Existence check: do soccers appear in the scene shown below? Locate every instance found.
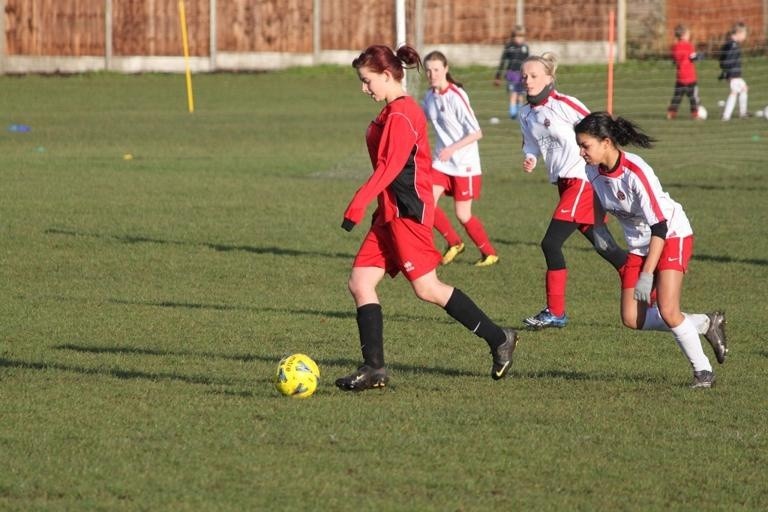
[763,105,768,118]
[274,354,321,397]
[698,105,707,119]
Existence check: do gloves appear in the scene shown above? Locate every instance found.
[632,272,653,305]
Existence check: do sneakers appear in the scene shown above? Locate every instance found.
[473,255,499,269]
[440,242,464,266]
[489,327,520,380]
[524,308,568,329]
[336,367,389,393]
[691,370,716,388]
[704,310,728,364]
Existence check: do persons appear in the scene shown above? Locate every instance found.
[333,44,520,390]
[574,111,727,389]
[416,50,500,266]
[717,22,755,119]
[494,26,529,119]
[666,22,701,119]
[519,53,628,328]
